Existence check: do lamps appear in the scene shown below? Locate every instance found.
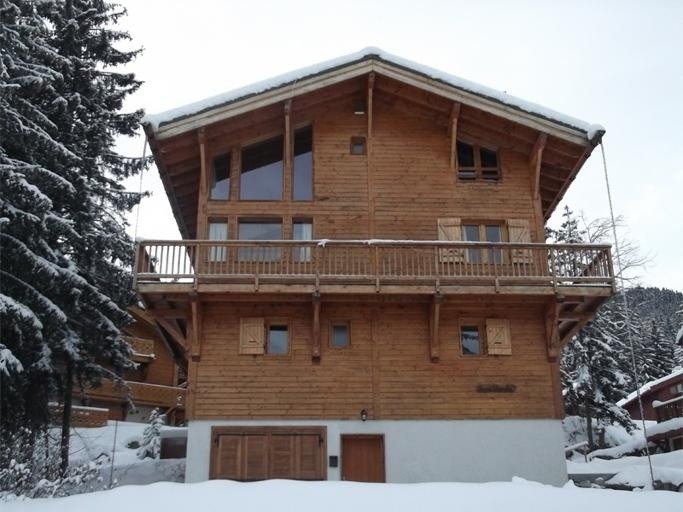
[360,409,369,422]
[312,289,320,301]
[351,78,365,115]
